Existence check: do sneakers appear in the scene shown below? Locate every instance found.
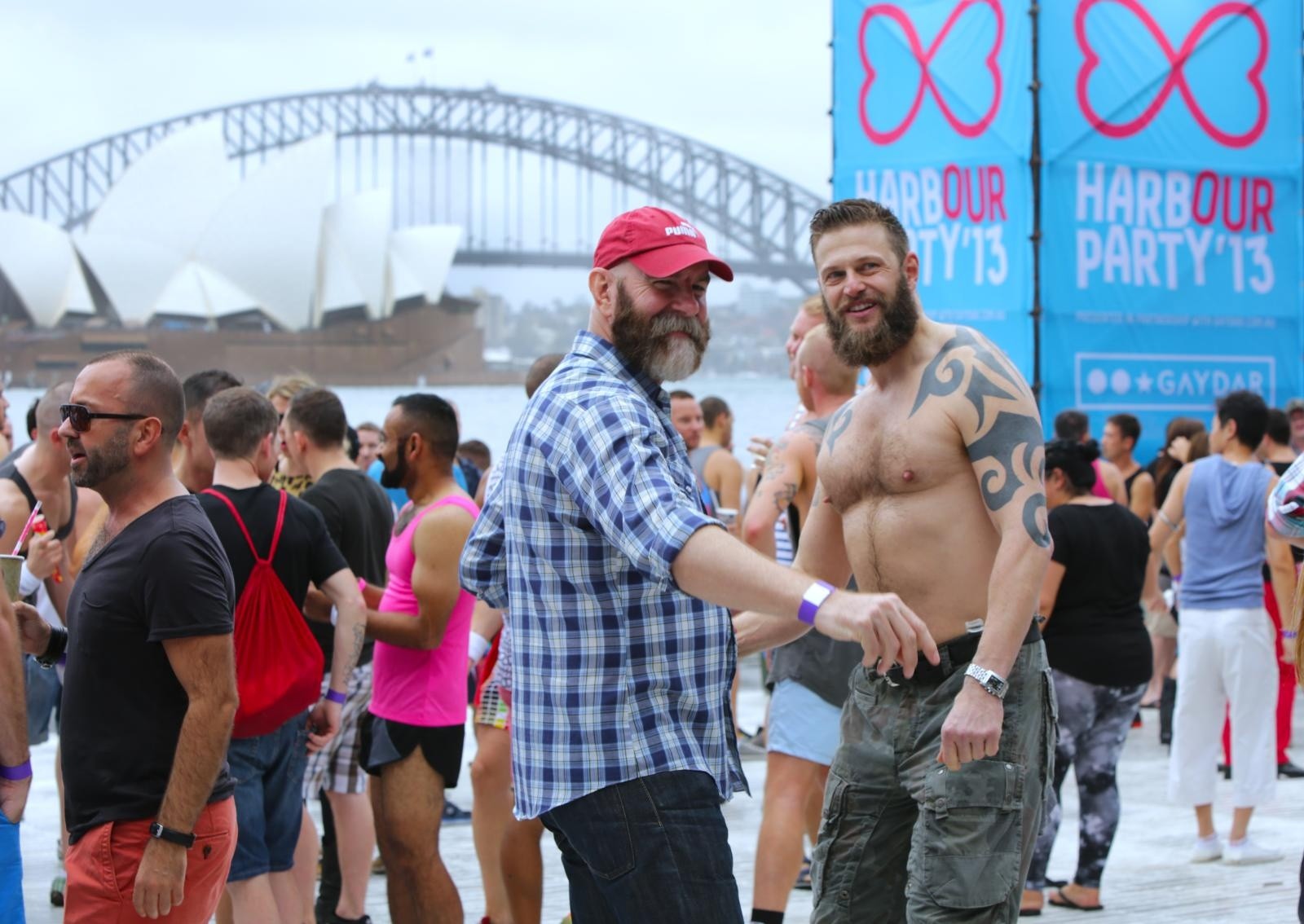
[1223,843,1285,865]
[1190,840,1223,861]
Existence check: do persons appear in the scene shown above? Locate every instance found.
[1053,392,1304,924]
[0,352,491,924]
[741,295,865,923]
[730,197,1059,924]
[459,206,941,924]
[1020,438,1153,916]
[469,354,745,924]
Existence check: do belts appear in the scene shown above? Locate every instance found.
[870,618,1044,688]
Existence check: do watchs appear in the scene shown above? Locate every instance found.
[797,578,837,627]
[964,663,1009,700]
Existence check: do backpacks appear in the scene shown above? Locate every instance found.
[198,487,323,737]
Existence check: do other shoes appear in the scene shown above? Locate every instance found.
[1278,764,1304,775]
[316,861,324,879]
[439,802,471,821]
[372,856,386,872]
[1218,765,1226,770]
[51,840,66,906]
[735,729,764,760]
[739,734,767,754]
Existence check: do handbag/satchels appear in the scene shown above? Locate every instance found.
[23,653,62,745]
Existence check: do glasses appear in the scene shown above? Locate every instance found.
[60,404,164,433]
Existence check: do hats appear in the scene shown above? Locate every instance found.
[1287,399,1304,415]
[594,206,734,282]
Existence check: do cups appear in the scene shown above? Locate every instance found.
[715,508,737,526]
[1,554,25,604]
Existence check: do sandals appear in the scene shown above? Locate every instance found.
[794,857,815,888]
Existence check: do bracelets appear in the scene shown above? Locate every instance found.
[326,687,346,702]
[330,599,338,627]
[1281,629,1297,639]
[1171,574,1181,580]
[3,759,32,780]
[358,575,367,592]
[35,618,66,669]
[148,821,197,850]
[18,560,42,595]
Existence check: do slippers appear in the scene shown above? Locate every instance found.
[1020,906,1040,914]
[1048,886,1103,909]
